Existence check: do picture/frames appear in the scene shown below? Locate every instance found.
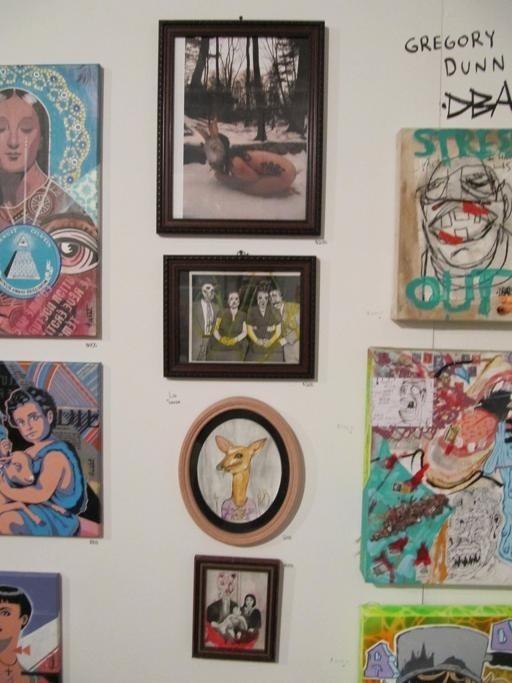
[163,254,318,382]
[156,18,325,236]
[177,396,306,548]
[191,555,283,663]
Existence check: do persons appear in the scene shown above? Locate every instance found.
[237,594,261,639]
[0,385,89,536]
[193,283,301,364]
[0,584,50,683]
[0,88,97,336]
[207,572,238,624]
